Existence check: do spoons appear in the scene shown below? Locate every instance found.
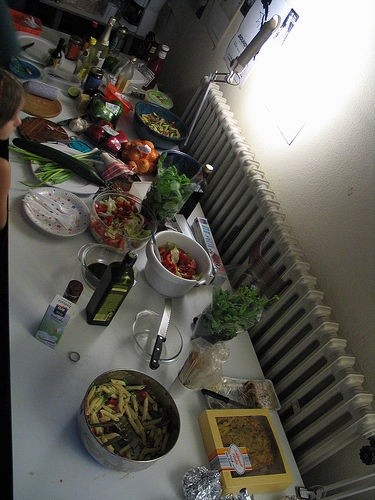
[15,56,33,76]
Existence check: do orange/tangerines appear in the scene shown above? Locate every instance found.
[120,140,160,174]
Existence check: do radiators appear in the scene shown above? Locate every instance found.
[177,76,374,473]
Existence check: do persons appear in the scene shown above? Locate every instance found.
[0,66,29,228]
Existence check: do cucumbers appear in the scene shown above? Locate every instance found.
[11,137,106,186]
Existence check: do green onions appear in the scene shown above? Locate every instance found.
[9,146,104,186]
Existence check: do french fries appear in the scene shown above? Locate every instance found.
[86,379,171,460]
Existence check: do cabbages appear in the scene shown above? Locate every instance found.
[95,195,152,242]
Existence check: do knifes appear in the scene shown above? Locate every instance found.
[201,386,253,408]
[148,294,173,368]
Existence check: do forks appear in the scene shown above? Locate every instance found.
[23,186,79,232]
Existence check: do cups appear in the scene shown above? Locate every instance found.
[88,65,104,93]
[171,337,230,392]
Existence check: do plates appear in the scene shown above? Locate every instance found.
[20,78,86,126]
[201,378,281,408]
[16,30,65,68]
[24,187,91,237]
[31,132,100,195]
[7,56,41,81]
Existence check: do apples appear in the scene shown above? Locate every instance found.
[87,119,128,152]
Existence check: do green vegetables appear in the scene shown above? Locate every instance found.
[196,285,280,345]
[143,156,191,221]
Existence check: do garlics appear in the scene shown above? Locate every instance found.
[69,118,90,131]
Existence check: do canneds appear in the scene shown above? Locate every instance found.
[84,66,105,96]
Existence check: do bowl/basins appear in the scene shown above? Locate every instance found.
[129,309,185,363]
[77,366,183,472]
[77,241,141,291]
[144,229,212,299]
[159,148,206,184]
[14,9,45,37]
[46,62,81,87]
[95,188,159,251]
[143,86,172,109]
[135,101,193,149]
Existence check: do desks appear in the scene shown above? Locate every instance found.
[9,25,309,500]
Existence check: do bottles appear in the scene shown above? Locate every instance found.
[142,43,171,91]
[110,54,140,88]
[95,16,117,70]
[180,160,213,222]
[80,35,99,67]
[85,250,139,326]
[47,36,67,69]
